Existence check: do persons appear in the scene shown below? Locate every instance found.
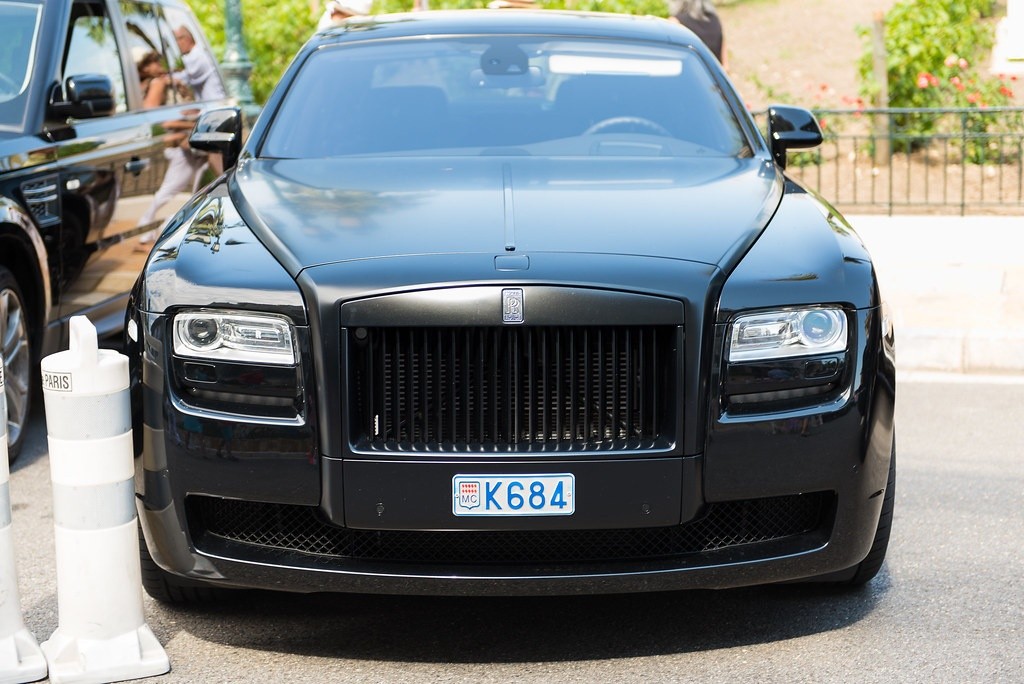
[670,0,723,65]
[137,26,244,245]
[317,0,373,29]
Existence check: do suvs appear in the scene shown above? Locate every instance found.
[0,0,242,471]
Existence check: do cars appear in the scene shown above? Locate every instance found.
[115,7,896,615]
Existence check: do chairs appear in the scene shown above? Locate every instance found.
[359,86,449,151]
[555,76,677,136]
